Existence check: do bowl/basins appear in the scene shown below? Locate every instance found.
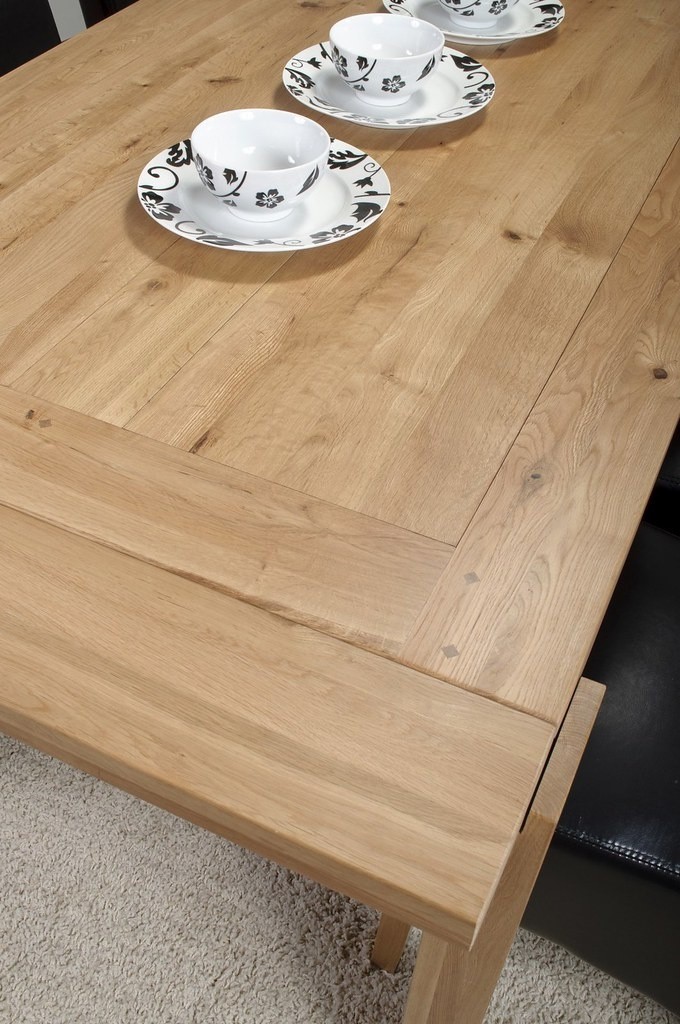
[328,14,445,107]
[438,0,512,29]
[192,108,330,222]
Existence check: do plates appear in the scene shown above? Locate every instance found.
[137,137,390,252]
[383,0,565,45]
[282,42,494,129]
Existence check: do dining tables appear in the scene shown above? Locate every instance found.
[0,0,680,1024]
